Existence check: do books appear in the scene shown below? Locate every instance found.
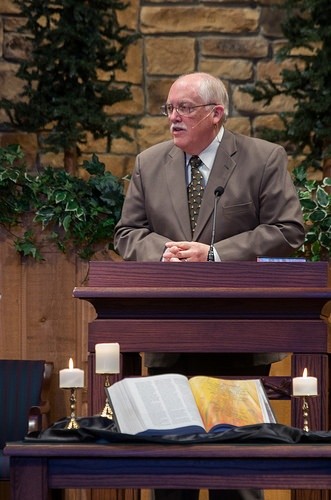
[104,372,277,434]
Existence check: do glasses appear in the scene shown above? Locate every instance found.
[159,102,218,116]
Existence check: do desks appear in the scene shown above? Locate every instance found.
[2,437,331,500]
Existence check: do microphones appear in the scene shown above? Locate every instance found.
[208,185,224,262]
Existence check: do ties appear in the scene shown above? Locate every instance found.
[186,155,204,237]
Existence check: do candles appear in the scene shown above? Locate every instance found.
[58,357,84,387]
[95,342,120,374]
[293,367,318,396]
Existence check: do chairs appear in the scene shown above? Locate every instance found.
[0,359,55,500]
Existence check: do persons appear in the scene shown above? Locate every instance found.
[113,72,306,500]
